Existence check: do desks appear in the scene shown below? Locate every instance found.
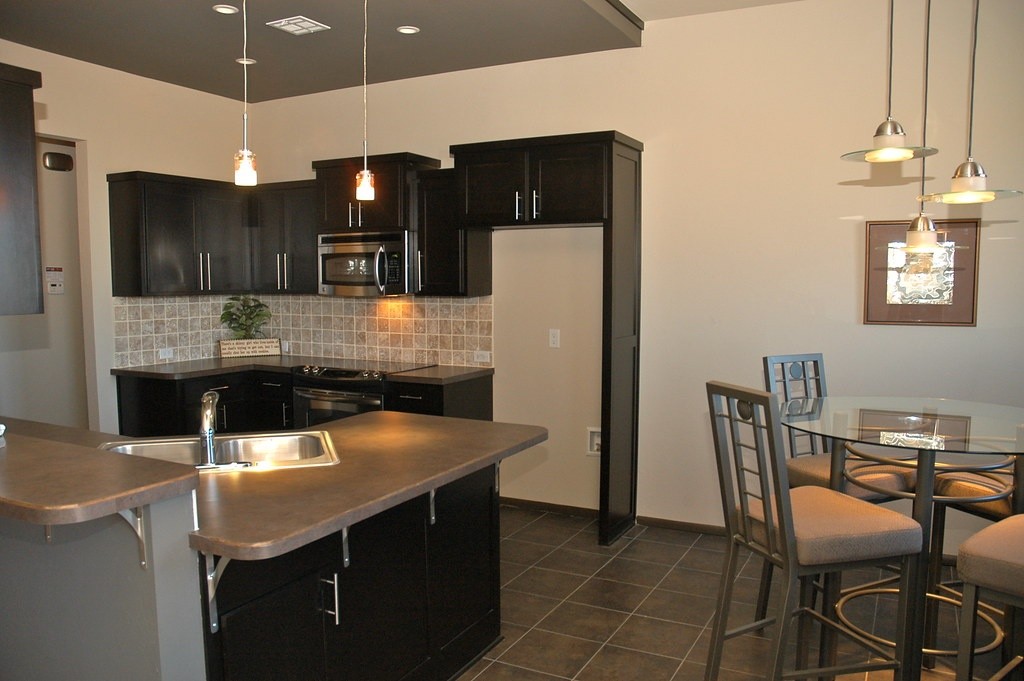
[777,396,1024,681]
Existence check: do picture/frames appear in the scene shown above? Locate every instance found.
[862,218,981,328]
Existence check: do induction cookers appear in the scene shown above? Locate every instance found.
[299,357,437,390]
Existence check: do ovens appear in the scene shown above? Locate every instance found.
[292,385,383,435]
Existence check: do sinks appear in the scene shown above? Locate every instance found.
[216,433,324,465]
[107,442,208,468]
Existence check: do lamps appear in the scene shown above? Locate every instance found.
[355,0,376,201]
[234,0,258,187]
[915,0,1024,205]
[840,0,938,164]
[875,0,970,254]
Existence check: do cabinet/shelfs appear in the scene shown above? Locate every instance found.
[248,179,317,294]
[0,61,44,315]
[116,371,255,437]
[385,374,493,422]
[256,370,295,431]
[312,152,441,234]
[448,130,644,230]
[199,460,505,681]
[415,168,492,299]
[107,171,250,297]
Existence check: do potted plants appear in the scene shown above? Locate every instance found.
[217,295,282,358]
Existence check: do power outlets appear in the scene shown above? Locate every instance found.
[283,342,289,352]
[549,328,560,348]
[160,348,173,360]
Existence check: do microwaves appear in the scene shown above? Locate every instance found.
[318,230,418,299]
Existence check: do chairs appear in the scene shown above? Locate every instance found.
[705,381,923,681]
[754,353,920,632]
[922,464,1015,670]
[956,512,1024,681]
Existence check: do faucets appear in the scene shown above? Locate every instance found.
[199,390,220,463]
